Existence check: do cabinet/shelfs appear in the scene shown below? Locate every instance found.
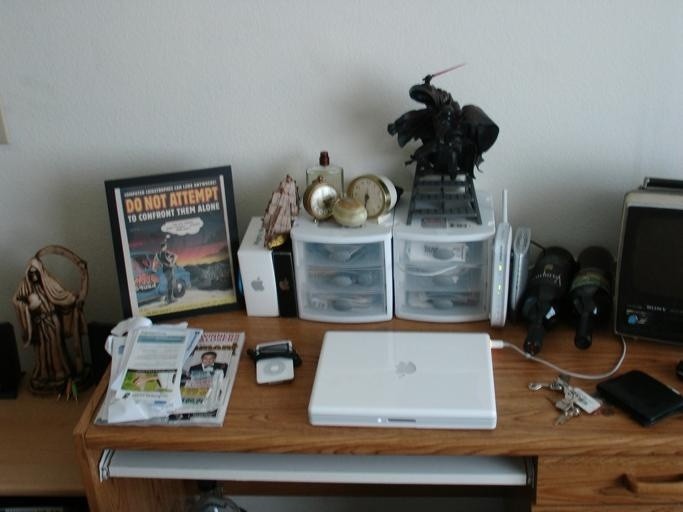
[291,204,391,322]
[393,189,491,323]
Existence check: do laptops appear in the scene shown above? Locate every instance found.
[308,330,497,430]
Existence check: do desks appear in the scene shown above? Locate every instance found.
[0,359,102,511]
[71,308,683,511]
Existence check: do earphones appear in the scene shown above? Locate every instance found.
[294,358,302,367]
[247,349,254,356]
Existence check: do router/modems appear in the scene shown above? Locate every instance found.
[489,189,512,328]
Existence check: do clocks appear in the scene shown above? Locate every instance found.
[346,175,398,217]
[303,174,338,222]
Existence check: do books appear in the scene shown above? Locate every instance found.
[97,327,245,427]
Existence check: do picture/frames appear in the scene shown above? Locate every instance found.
[105,165,243,322]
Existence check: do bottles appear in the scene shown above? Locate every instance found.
[570,246,619,350]
[306,152,344,199]
[523,246,577,356]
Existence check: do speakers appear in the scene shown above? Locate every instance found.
[0,322,22,400]
[613,188,683,348]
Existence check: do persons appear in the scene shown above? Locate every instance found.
[158,243,178,304]
[11,258,76,381]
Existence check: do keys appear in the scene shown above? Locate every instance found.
[545,374,605,424]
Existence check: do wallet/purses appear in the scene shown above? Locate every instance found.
[596,370,682,426]
